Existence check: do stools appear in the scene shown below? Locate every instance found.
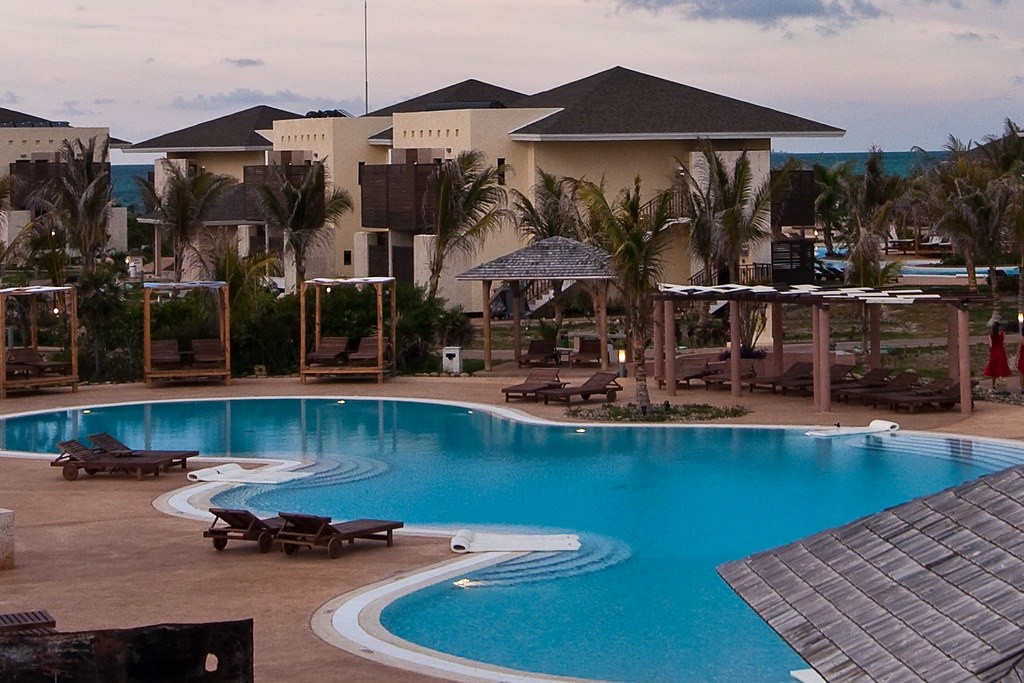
[0,610,56,632]
[0,627,57,634]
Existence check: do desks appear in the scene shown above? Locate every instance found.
[108,451,133,475]
[913,390,935,411]
[558,350,574,366]
[277,523,312,551]
[545,381,570,400]
[701,369,722,389]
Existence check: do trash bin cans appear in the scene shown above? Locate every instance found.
[442,346,463,373]
[129,265,137,278]
[560,330,568,348]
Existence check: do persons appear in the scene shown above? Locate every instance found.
[982,310,1013,390]
[1015,326,1024,395]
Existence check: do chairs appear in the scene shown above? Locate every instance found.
[814,266,903,283]
[151,340,180,370]
[502,367,561,402]
[986,270,1019,291]
[193,340,225,369]
[655,357,979,415]
[51,440,171,481]
[570,340,610,368]
[202,507,404,559]
[349,337,389,366]
[307,337,350,365]
[87,433,199,473]
[519,340,556,367]
[537,372,623,406]
[4,348,68,378]
